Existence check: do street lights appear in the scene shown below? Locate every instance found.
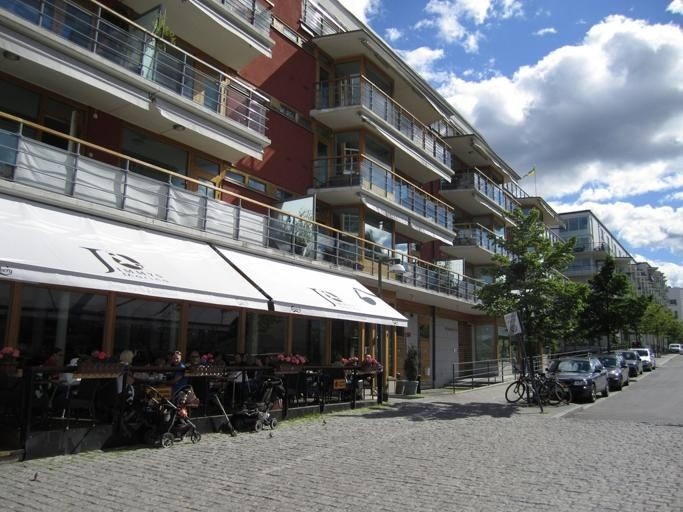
[377,257,405,404]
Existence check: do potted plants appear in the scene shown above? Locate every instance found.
[402,346,424,394]
[289,209,314,255]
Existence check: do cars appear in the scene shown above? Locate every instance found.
[670,344,680,353]
[552,347,655,403]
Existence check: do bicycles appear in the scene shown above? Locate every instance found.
[505,367,573,405]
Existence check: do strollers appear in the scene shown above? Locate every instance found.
[234,378,286,431]
[142,384,202,448]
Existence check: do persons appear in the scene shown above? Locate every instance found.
[24,338,272,414]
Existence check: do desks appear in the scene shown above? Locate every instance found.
[120,362,384,419]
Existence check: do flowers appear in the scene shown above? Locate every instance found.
[273,352,307,366]
[339,354,381,370]
